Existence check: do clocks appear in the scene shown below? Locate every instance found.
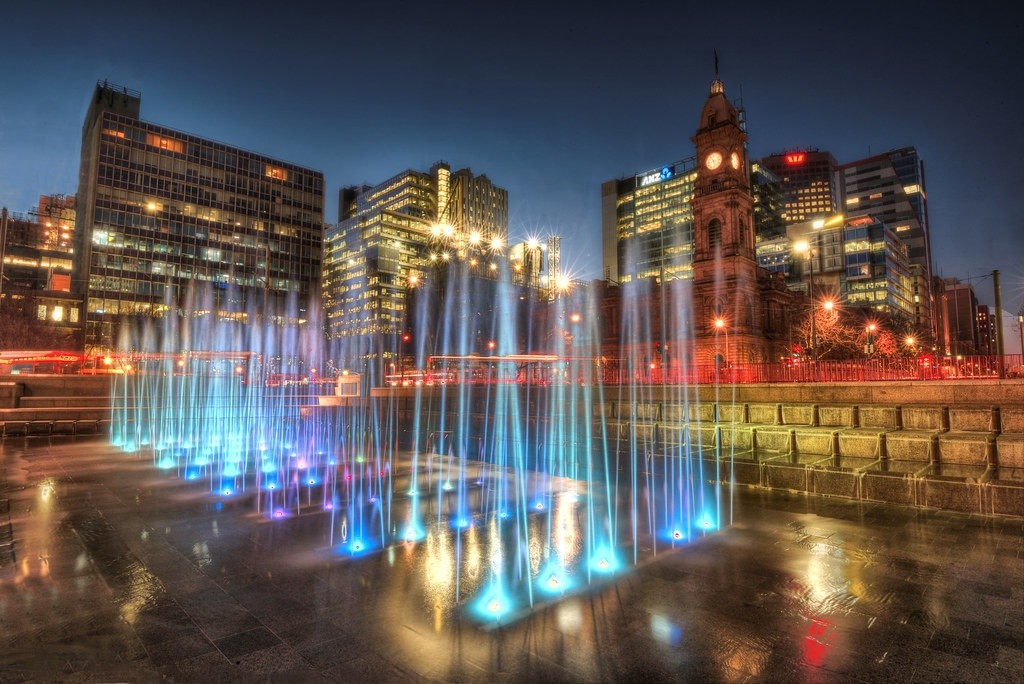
[729,153,740,169]
[704,153,722,172]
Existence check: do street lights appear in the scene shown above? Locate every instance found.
[824,302,833,319]
[869,324,875,360]
[794,241,815,372]
[571,314,580,356]
[390,364,395,383]
[527,238,540,303]
[908,338,914,364]
[401,277,417,384]
[715,320,729,371]
